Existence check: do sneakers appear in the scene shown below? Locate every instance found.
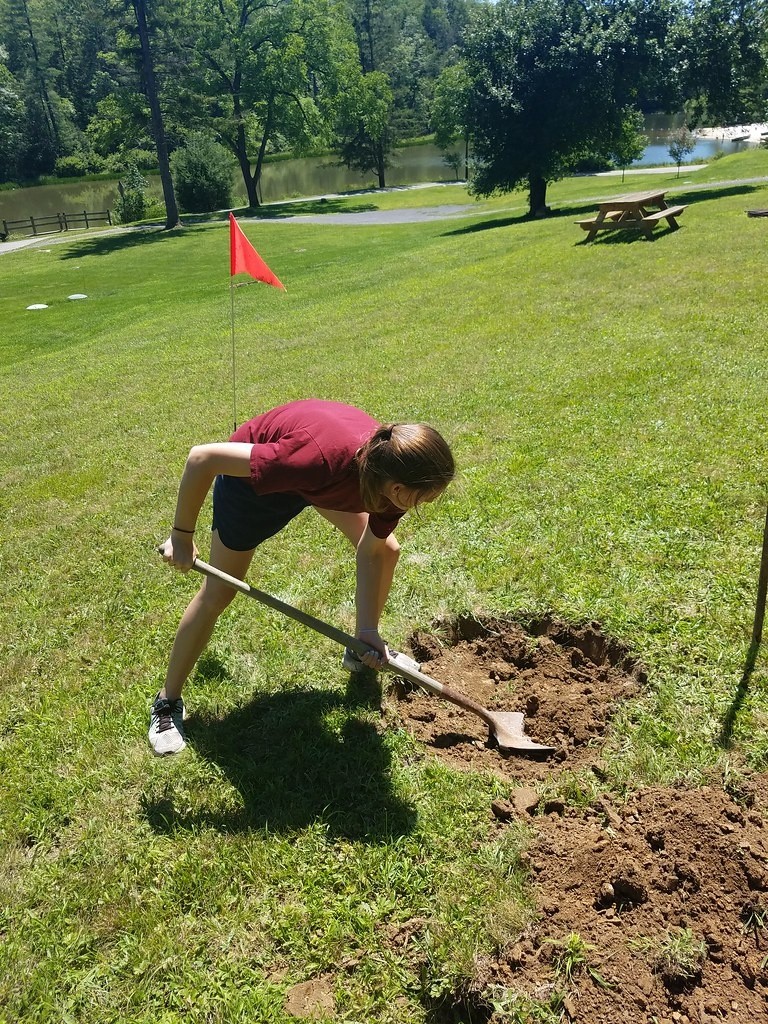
[342,643,422,673]
[148,691,187,756]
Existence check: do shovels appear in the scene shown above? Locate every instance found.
[158,538,560,754]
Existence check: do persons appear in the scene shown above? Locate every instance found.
[148,399,458,756]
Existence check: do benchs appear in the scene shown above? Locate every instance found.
[640,203,690,228]
[573,211,624,231]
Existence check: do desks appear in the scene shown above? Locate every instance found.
[586,190,669,244]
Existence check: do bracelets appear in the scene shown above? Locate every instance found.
[170,527,196,533]
[358,629,378,633]
